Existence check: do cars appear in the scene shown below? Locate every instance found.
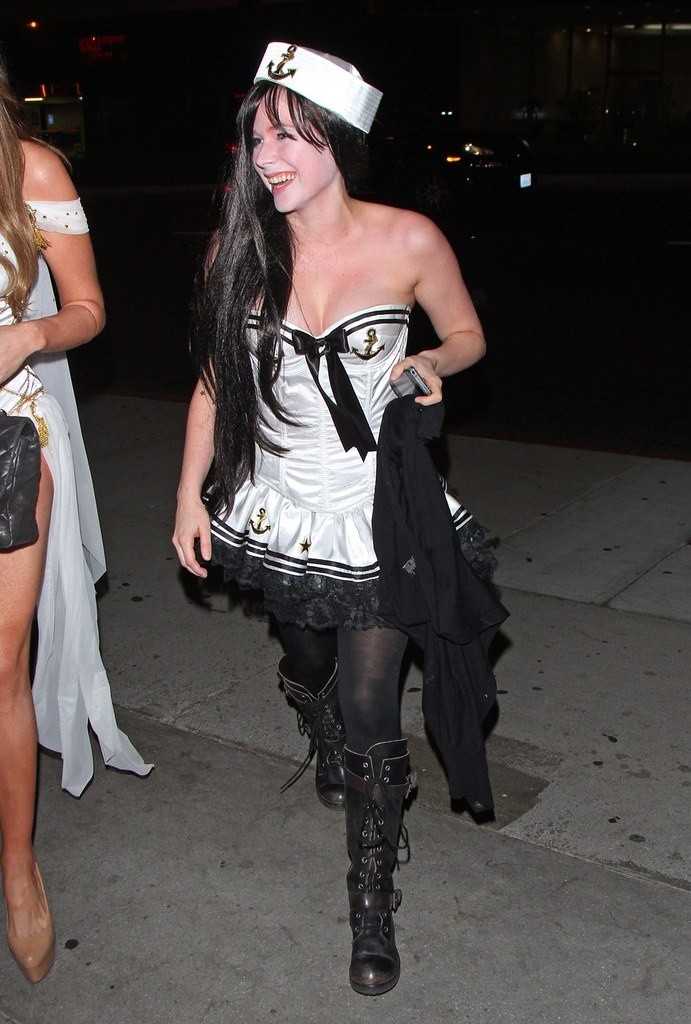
[339,91,541,219]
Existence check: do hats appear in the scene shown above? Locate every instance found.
[251,40,384,134]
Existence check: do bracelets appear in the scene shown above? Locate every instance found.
[58,304,98,346]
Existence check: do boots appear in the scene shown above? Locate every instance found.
[341,737,420,997]
[276,653,346,813]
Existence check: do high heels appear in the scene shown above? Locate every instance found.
[3,860,57,983]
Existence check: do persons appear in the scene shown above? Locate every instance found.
[0,71,110,983]
[168,40,488,998]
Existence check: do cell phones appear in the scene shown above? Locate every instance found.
[391,365,431,397]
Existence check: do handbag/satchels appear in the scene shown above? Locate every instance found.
[0,409,42,549]
